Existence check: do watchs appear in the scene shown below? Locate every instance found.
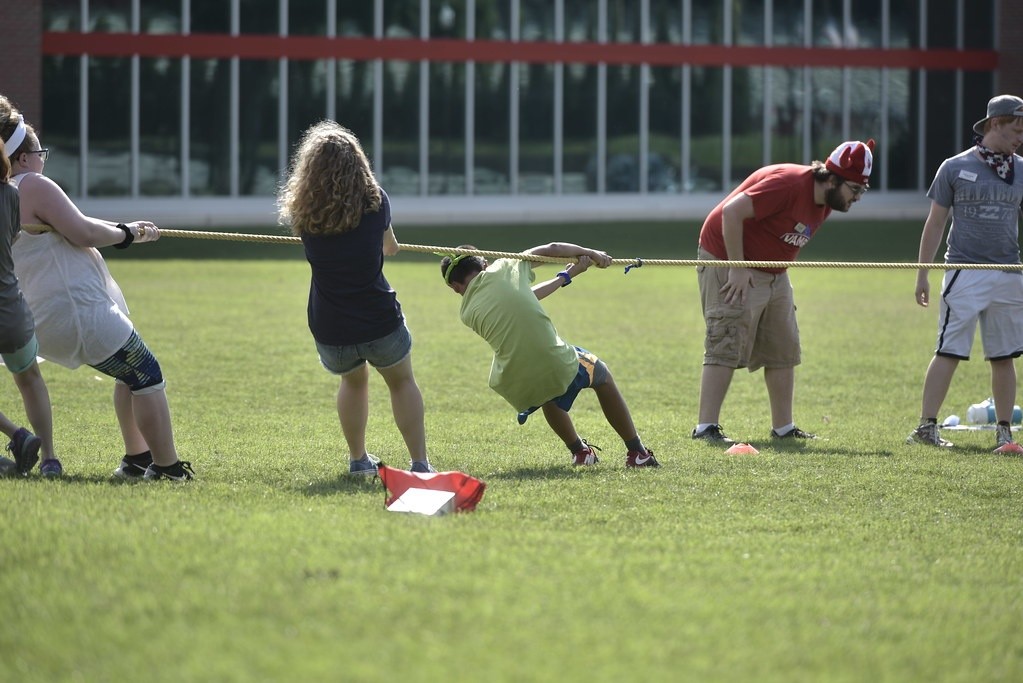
[557,271,573,288]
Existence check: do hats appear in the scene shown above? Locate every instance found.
[973,94,1023,136]
[825,139,875,185]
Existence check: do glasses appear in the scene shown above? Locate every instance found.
[16,148,49,160]
[845,182,868,195]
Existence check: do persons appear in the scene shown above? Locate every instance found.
[691,140,876,442]
[277,122,436,480]
[0,95,194,483]
[443,243,661,471]
[907,95,1023,448]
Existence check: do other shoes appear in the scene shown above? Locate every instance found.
[410,462,439,473]
[5,427,42,474]
[38,458,62,477]
[350,453,380,474]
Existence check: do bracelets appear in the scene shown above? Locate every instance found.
[113,223,134,250]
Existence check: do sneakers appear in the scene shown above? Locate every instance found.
[770,426,829,441]
[626,446,662,468]
[996,420,1013,446]
[692,424,735,443]
[573,439,602,465]
[906,417,953,447]
[114,458,195,481]
